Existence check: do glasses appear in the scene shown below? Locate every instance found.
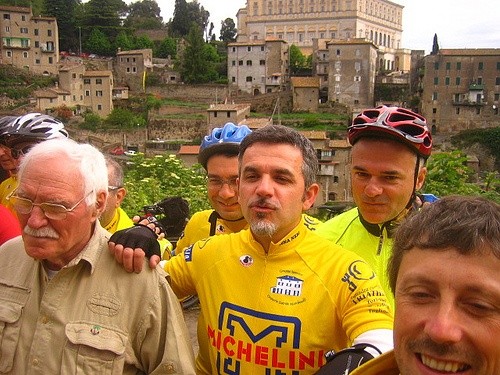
[11,145,31,158]
[6,188,93,220]
[108,186,122,192]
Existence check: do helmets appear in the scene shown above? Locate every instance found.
[196,123,254,170]
[8,113,69,143]
[348,105,432,159]
[0,116,17,134]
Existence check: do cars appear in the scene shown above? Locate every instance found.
[110,148,123,154]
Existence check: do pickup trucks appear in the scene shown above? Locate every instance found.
[125,150,138,155]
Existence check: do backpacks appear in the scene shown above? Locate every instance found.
[144,196,190,226]
